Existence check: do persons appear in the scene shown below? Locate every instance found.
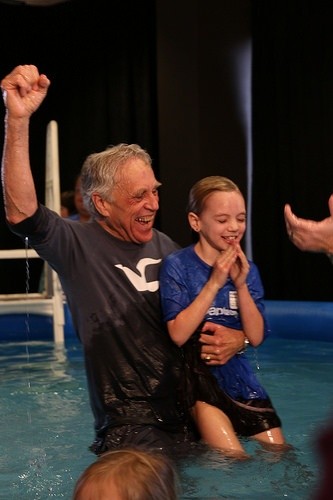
[37,193,76,334]
[0,65,251,434]
[284,194,333,254]
[160,176,286,449]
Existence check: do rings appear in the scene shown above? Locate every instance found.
[290,232,293,240]
[204,356,210,362]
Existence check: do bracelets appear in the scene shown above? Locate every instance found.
[237,337,249,354]
